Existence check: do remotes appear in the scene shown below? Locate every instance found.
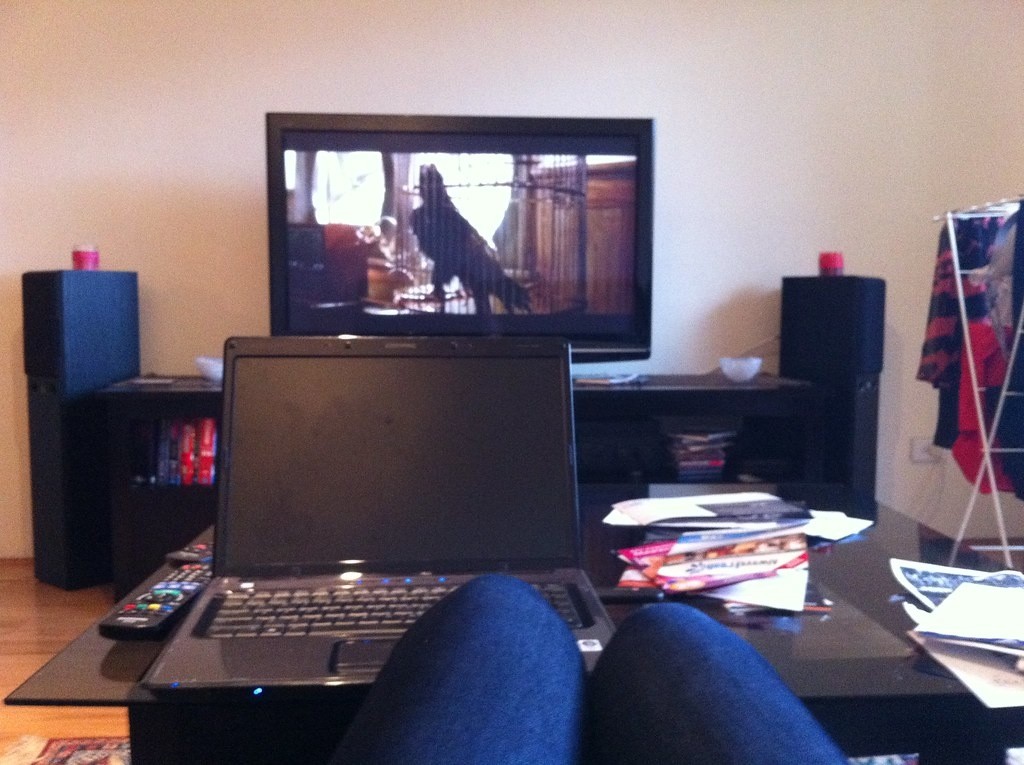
[98,538,215,643]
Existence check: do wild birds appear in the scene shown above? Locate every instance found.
[409,164,534,314]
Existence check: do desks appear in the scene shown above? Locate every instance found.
[0,481,1024,765]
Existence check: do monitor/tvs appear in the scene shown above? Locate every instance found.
[262,109,651,363]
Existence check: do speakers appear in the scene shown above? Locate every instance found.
[19,270,140,590]
[776,277,887,510]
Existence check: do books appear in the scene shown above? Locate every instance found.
[656,417,738,483]
[889,558,1024,709]
[604,493,876,616]
[572,373,638,385]
[154,417,219,485]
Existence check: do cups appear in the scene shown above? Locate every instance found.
[72,245,101,272]
[818,250,844,277]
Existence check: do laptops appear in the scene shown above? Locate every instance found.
[146,335,616,703]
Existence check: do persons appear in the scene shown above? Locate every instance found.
[326,572,852,765]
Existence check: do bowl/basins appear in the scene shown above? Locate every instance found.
[196,356,224,383]
[720,356,763,384]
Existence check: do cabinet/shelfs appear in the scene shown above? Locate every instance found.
[73,374,812,600]
